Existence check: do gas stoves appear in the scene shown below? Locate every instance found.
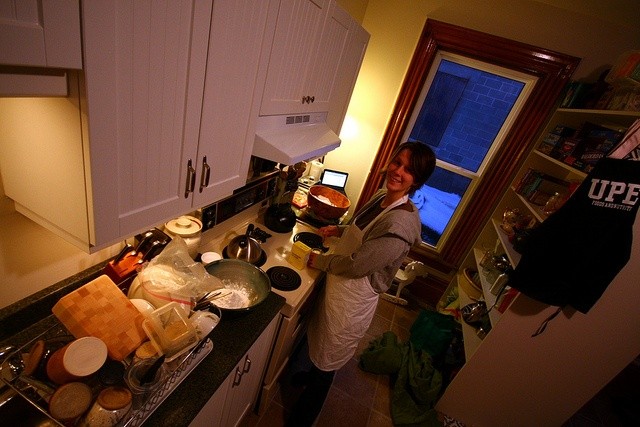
[202,179,340,307]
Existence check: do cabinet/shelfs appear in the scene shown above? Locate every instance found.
[258,283,318,416]
[2,0,84,97]
[81,0,271,248]
[188,316,279,427]
[259,0,354,116]
[435,111,640,427]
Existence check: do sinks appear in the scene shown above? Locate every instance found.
[1,386,49,426]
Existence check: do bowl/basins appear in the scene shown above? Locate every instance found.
[308,185,351,219]
[459,267,482,299]
[203,259,271,312]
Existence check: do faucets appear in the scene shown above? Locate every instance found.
[0,345,26,375]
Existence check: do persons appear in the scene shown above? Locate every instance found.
[284,141,436,426]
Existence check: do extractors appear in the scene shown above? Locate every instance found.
[251,111,342,166]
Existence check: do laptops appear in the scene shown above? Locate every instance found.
[322,168,349,198]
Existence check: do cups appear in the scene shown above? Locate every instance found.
[308,247,322,269]
[201,251,221,265]
[190,310,220,340]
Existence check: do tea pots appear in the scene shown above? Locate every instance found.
[227,224,263,263]
[263,191,296,233]
[461,301,486,324]
[502,206,523,232]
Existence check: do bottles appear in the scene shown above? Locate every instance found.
[479,250,495,266]
[482,256,497,275]
[486,262,507,284]
[489,271,508,296]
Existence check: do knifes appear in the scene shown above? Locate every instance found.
[113,232,160,267]
